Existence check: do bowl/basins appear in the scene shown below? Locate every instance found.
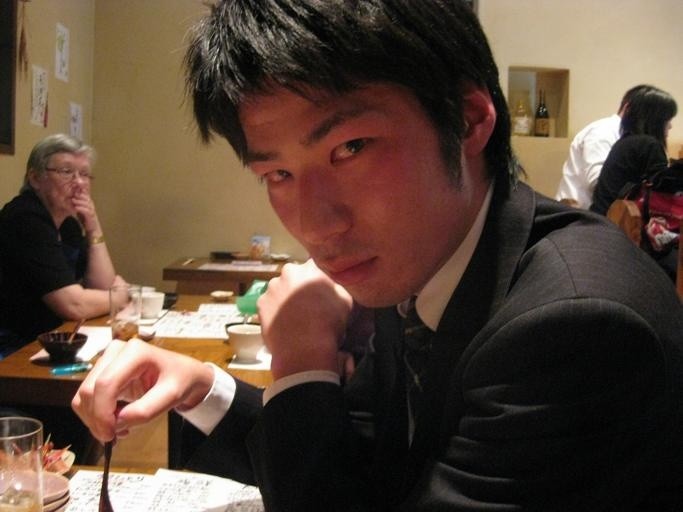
[210,290,234,302]
[163,293,178,308]
[37,333,87,362]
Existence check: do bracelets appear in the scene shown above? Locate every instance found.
[86,235,108,246]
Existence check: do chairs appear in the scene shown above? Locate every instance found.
[607,198,642,248]
[674,227,683,299]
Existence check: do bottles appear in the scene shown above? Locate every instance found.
[511,88,532,136]
[533,89,550,137]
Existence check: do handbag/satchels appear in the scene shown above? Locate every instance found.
[636,176,682,251]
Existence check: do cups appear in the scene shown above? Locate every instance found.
[0,417,44,512]
[227,322,262,364]
[133,292,165,318]
[140,286,155,293]
[111,285,142,340]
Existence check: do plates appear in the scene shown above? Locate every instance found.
[272,254,291,261]
[17,449,76,475]
[44,490,70,512]
[0,469,70,505]
[52,497,69,512]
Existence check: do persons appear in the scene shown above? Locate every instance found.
[590,89,682,218]
[557,86,658,208]
[0,136,130,462]
[70,1,681,511]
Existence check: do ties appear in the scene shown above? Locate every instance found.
[400,299,429,455]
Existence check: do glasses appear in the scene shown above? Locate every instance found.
[41,165,96,187]
